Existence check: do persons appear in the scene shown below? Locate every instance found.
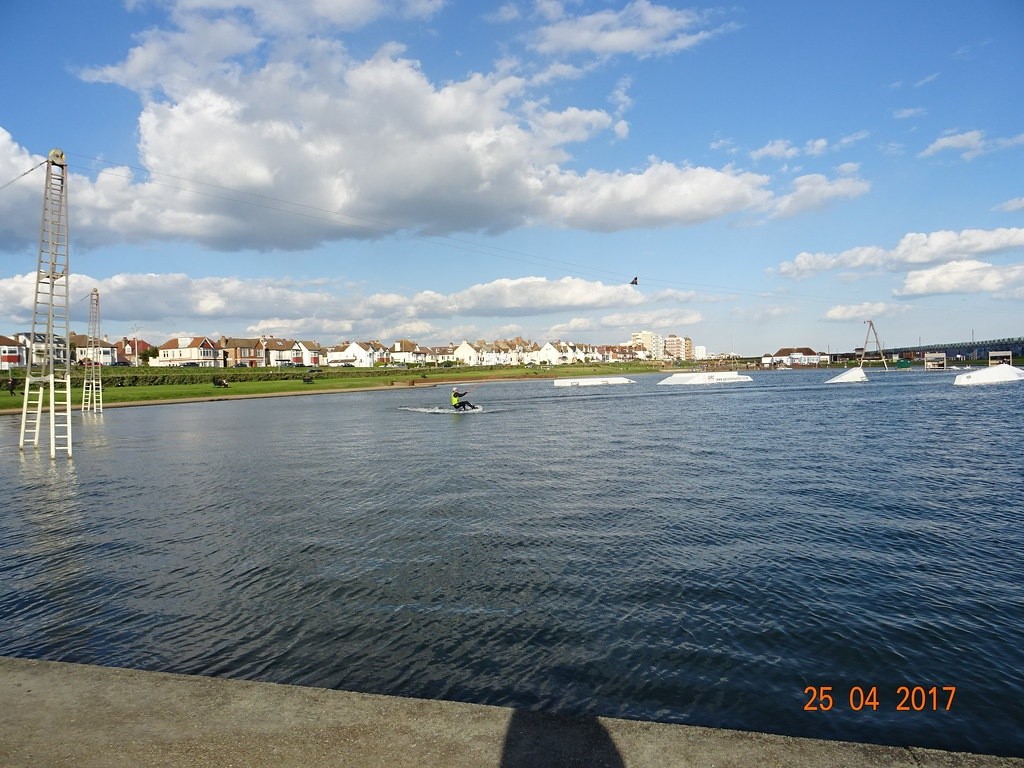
[451,387,478,411]
[223,378,229,388]
[7,378,16,396]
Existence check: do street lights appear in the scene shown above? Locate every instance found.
[402,336,411,357]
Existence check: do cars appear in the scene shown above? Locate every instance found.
[84,362,103,366]
[108,361,129,366]
[179,363,199,368]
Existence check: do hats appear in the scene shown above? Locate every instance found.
[452,387,458,391]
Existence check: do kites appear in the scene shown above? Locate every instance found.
[630,277,637,285]
